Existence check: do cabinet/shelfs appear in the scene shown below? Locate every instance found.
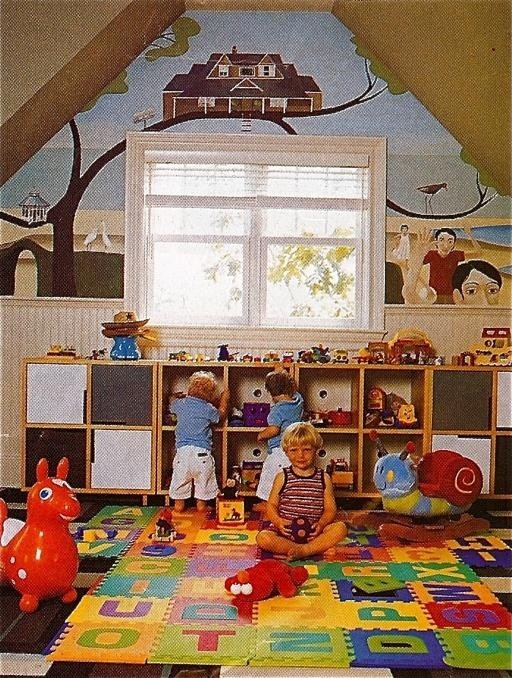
[18,357,512,508]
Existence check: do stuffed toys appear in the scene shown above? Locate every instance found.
[352,428,493,543]
[223,559,310,604]
[163,391,187,426]
[294,512,314,540]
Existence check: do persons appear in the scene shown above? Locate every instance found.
[256,420,347,561]
[402,226,502,305]
[166,371,230,515]
[390,224,413,271]
[424,226,486,305]
[256,370,305,502]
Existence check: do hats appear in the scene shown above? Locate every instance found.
[101,312,150,337]
